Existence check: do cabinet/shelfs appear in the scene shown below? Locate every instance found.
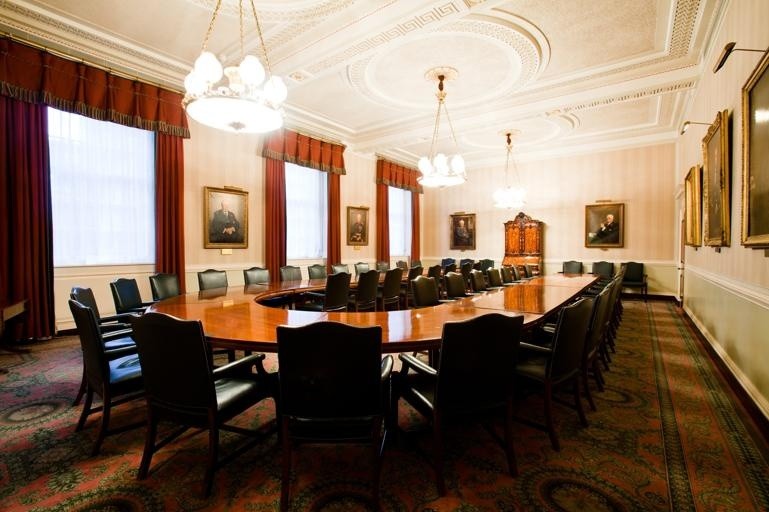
[500,213,545,278]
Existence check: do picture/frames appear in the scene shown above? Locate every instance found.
[446,210,476,253]
[681,46,769,260]
[582,200,626,251]
[201,185,250,251]
[345,204,371,247]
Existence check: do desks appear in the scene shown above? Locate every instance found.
[150,284,542,469]
[260,271,463,307]
[411,274,604,370]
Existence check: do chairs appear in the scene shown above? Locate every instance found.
[108,278,157,322]
[197,269,228,290]
[468,269,499,294]
[523,265,533,279]
[391,313,522,499]
[479,259,494,268]
[148,273,179,301]
[276,322,393,511]
[426,265,441,288]
[69,285,130,405]
[377,268,404,312]
[460,259,474,268]
[544,288,611,414]
[69,300,149,452]
[460,262,471,293]
[128,313,282,487]
[486,267,512,288]
[513,266,522,280]
[442,257,456,269]
[399,265,424,310]
[299,272,352,312]
[446,272,481,300]
[243,267,283,308]
[569,264,628,365]
[501,266,525,284]
[410,275,453,310]
[469,263,480,292]
[308,264,326,279]
[511,299,595,454]
[440,263,456,298]
[587,261,615,278]
[558,260,582,273]
[620,263,648,304]
[279,265,306,309]
[348,269,381,313]
[354,261,421,274]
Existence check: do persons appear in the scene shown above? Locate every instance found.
[592,212,618,243]
[456,220,469,245]
[209,197,240,243]
[350,212,364,240]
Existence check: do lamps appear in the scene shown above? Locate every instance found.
[712,41,767,73]
[490,128,529,212]
[181,0,288,138]
[414,64,467,188]
